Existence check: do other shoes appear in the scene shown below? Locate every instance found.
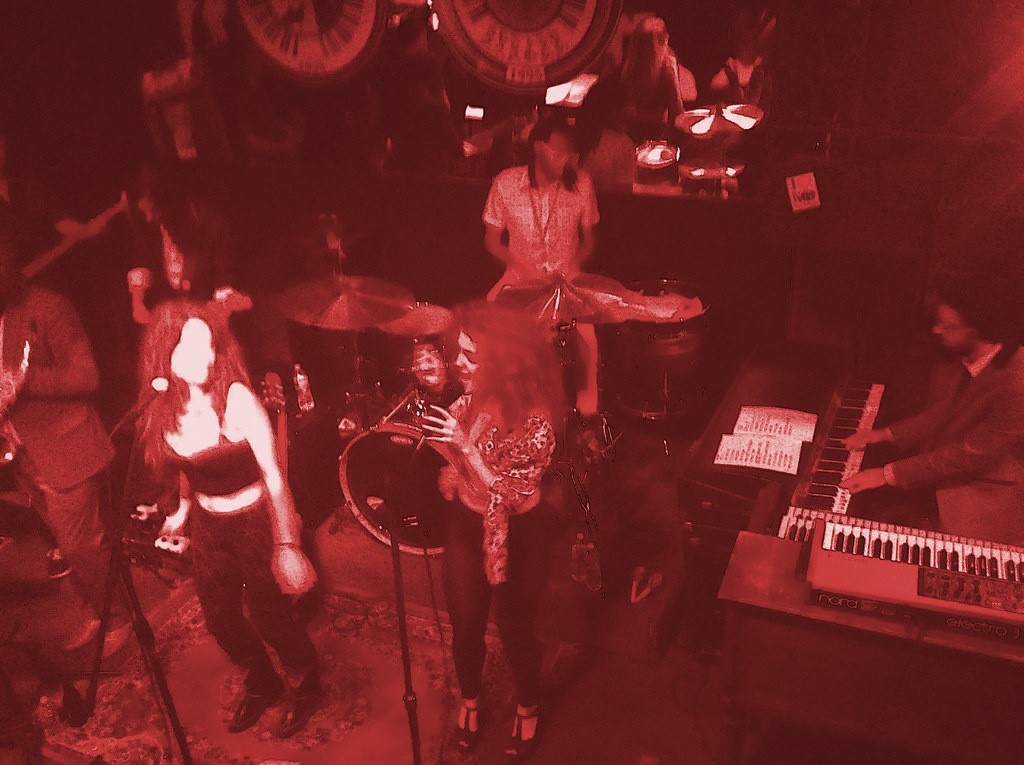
[101,623,131,660]
[62,618,101,652]
[230,674,284,732]
[276,685,324,738]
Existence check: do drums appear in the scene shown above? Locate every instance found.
[332,385,483,561]
[380,301,461,351]
[634,137,747,208]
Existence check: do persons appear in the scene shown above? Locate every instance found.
[425,297,565,748]
[131,297,321,741]
[604,20,685,145]
[485,111,601,426]
[837,267,1024,557]
[2,226,132,660]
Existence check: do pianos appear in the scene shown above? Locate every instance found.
[776,376,1024,644]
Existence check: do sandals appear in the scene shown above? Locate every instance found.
[503,700,542,757]
[454,694,485,753]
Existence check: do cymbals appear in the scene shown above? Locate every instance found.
[271,276,415,333]
[672,103,762,138]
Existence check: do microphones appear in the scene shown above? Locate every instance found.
[111,377,169,433]
[416,379,464,451]
